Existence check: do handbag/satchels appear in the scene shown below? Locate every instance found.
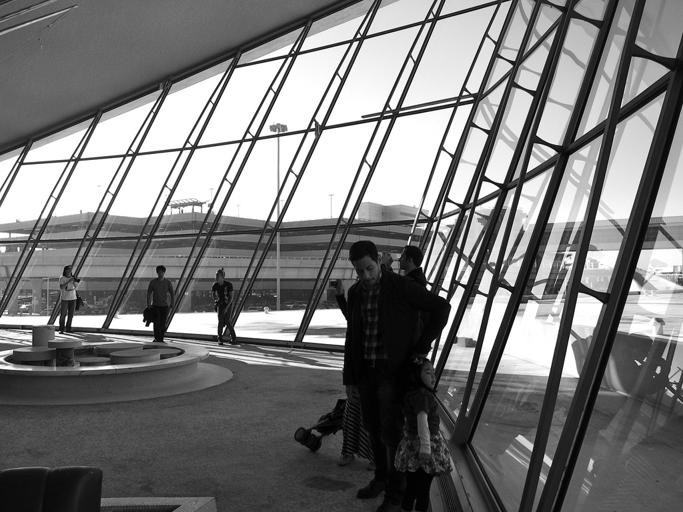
[75,290,85,314]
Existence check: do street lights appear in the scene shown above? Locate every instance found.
[268,124,288,313]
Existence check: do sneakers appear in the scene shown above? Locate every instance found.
[337,449,353,467]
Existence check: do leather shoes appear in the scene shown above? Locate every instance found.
[357,480,388,500]
[378,497,403,511]
[231,339,236,343]
[217,336,225,345]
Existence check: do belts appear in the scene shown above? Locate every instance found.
[151,305,171,309]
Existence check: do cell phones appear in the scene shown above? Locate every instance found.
[328,280,340,288]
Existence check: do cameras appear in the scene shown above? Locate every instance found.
[71,275,81,283]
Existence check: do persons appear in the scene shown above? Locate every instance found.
[349,240,452,512]
[211,269,237,346]
[330,278,377,471]
[394,356,453,512]
[59,265,80,334]
[380,245,427,288]
[146,265,175,344]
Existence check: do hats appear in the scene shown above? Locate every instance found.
[65,264,73,269]
